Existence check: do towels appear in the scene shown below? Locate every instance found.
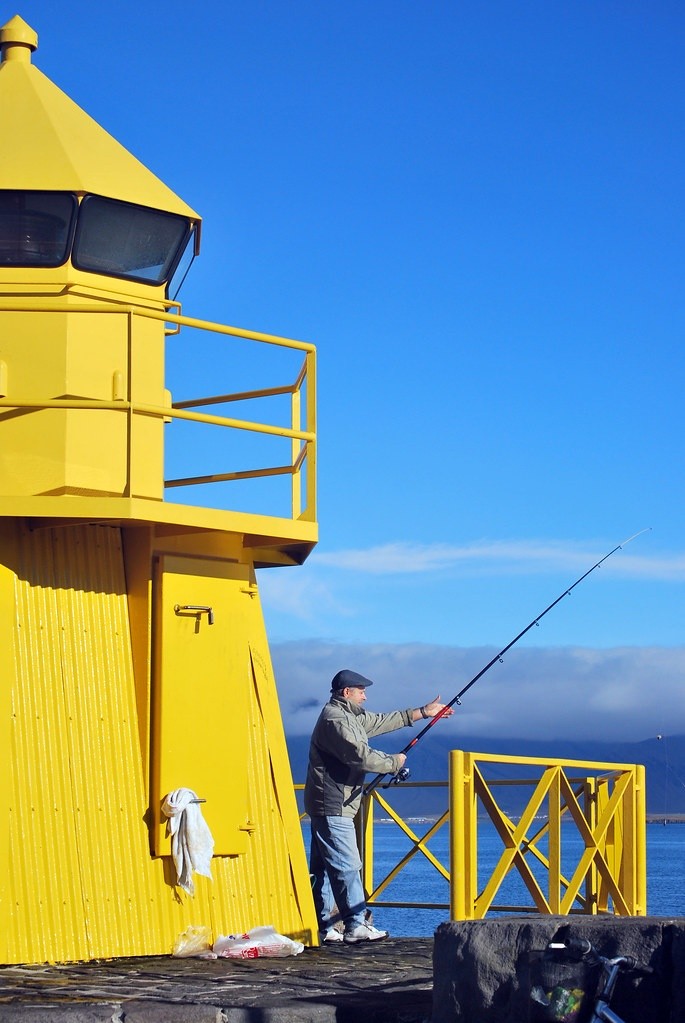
[161,787,217,900]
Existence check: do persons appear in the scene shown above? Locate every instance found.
[304,669,454,944]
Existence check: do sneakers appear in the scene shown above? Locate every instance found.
[319,927,344,944]
[344,922,390,944]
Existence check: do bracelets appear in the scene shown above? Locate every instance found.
[420,706,428,719]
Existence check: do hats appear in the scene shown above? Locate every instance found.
[332,670,373,690]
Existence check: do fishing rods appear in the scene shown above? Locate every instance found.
[362,525,655,796]
